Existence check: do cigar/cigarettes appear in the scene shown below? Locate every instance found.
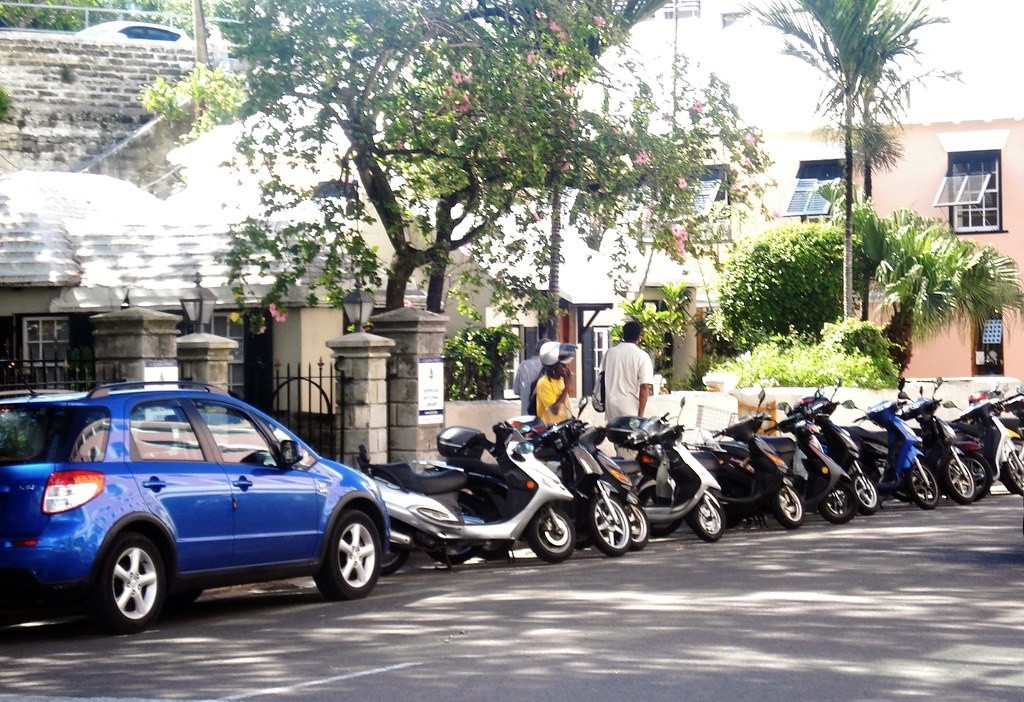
[569,372,574,374]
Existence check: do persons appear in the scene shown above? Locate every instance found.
[986,345,1003,365]
[599,322,653,460]
[536,341,574,426]
[514,338,553,416]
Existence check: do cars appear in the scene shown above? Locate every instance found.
[0,380,390,630]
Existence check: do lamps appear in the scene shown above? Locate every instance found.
[178,271,218,334]
[341,282,377,332]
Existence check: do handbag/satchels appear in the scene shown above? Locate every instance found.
[527,380,536,416]
[591,349,609,412]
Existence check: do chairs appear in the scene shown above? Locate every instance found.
[139,423,174,460]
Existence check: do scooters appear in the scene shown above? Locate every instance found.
[357,376,1024,573]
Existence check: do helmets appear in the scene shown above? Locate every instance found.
[539,342,579,365]
